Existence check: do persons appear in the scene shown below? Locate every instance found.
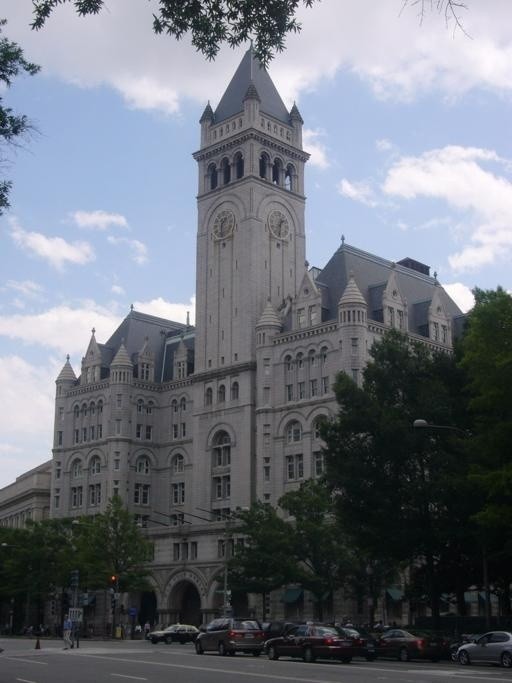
[337,617,400,632]
[70,616,83,649]
[118,618,151,640]
[62,612,74,650]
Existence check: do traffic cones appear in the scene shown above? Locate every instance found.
[34,640,41,649]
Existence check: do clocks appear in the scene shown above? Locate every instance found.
[268,210,290,240]
[211,210,235,240]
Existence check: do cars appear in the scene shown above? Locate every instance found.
[450,631,512,666]
[195,617,447,662]
[147,624,200,645]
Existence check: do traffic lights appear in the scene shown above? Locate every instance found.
[111,575,117,585]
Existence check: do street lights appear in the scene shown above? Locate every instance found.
[413,420,491,631]
